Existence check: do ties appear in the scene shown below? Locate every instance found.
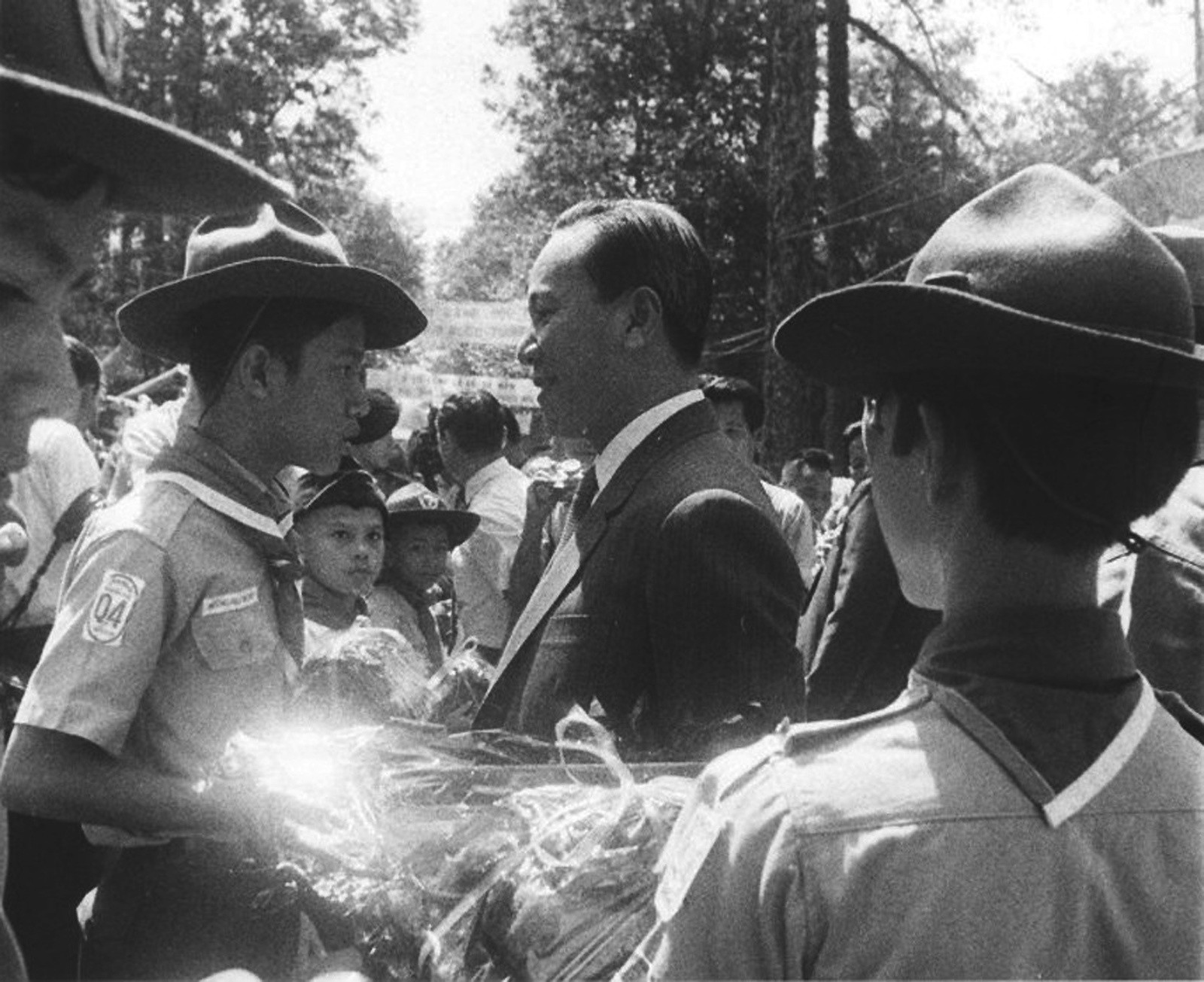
[544,467,599,576]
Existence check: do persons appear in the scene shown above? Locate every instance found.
[369,481,480,679]
[294,467,390,650]
[0,0,299,979]
[613,160,1203,982]
[0,197,430,982]
[470,199,808,766]
[316,388,402,490]
[1096,224,1203,746]
[775,417,944,722]
[0,333,186,982]
[697,375,816,613]
[405,388,598,718]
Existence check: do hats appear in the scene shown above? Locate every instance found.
[0,0,295,217]
[114,199,427,364]
[384,482,481,549]
[291,453,390,521]
[774,166,1204,465]
[344,389,400,445]
